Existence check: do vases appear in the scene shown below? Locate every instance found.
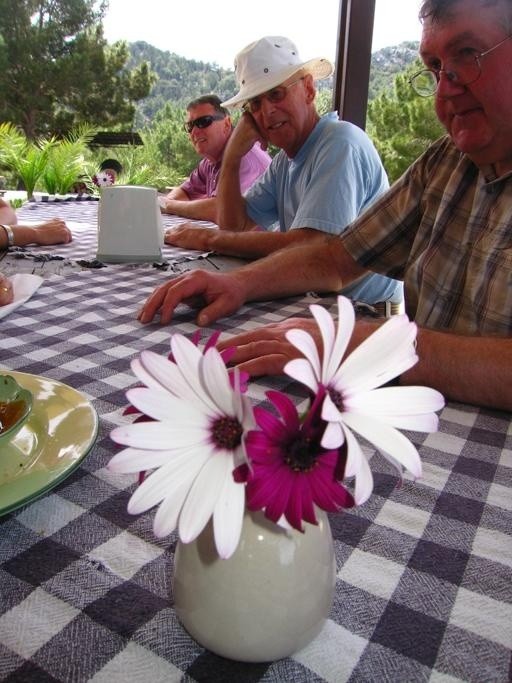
[174,505,337,665]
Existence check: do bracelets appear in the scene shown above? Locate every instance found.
[0,224,14,246]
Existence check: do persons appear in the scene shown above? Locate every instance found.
[158,95,273,230]
[0,218,72,250]
[0,273,14,306]
[164,36,407,318]
[136,0,512,413]
[0,199,17,225]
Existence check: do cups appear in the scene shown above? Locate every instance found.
[97,184,162,264]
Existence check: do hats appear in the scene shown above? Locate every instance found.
[220,36,333,108]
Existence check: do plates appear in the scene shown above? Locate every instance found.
[1,368,100,517]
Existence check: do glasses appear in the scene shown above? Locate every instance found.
[184,115,224,133]
[242,77,304,113]
[408,35,512,97]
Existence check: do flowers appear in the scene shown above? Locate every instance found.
[101,294,448,563]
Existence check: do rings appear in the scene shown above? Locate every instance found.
[3,288,7,293]
